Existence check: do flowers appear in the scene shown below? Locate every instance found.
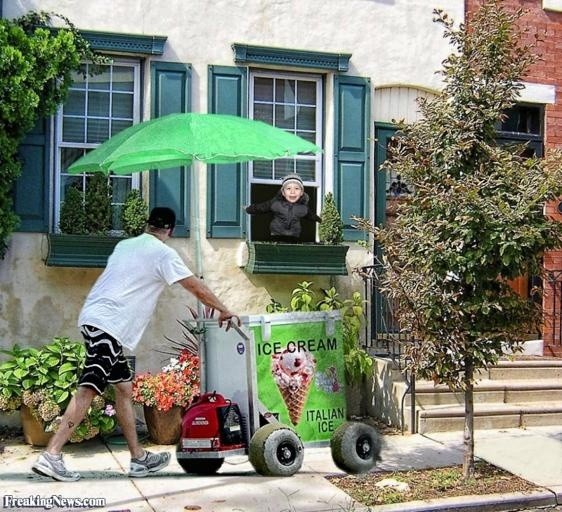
[0,336,201,445]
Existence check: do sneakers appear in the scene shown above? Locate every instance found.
[128,450,170,477]
[32,452,81,481]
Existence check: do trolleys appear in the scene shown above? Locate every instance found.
[175,316,382,478]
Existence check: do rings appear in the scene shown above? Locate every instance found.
[238,317,241,320]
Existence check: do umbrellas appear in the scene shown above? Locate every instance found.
[67,113,325,393]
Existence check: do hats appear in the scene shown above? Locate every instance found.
[281,174,304,195]
[146,207,176,229]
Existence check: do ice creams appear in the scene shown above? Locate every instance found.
[271,345,316,428]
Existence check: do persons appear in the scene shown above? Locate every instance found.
[243,174,322,242]
[32,207,242,482]
